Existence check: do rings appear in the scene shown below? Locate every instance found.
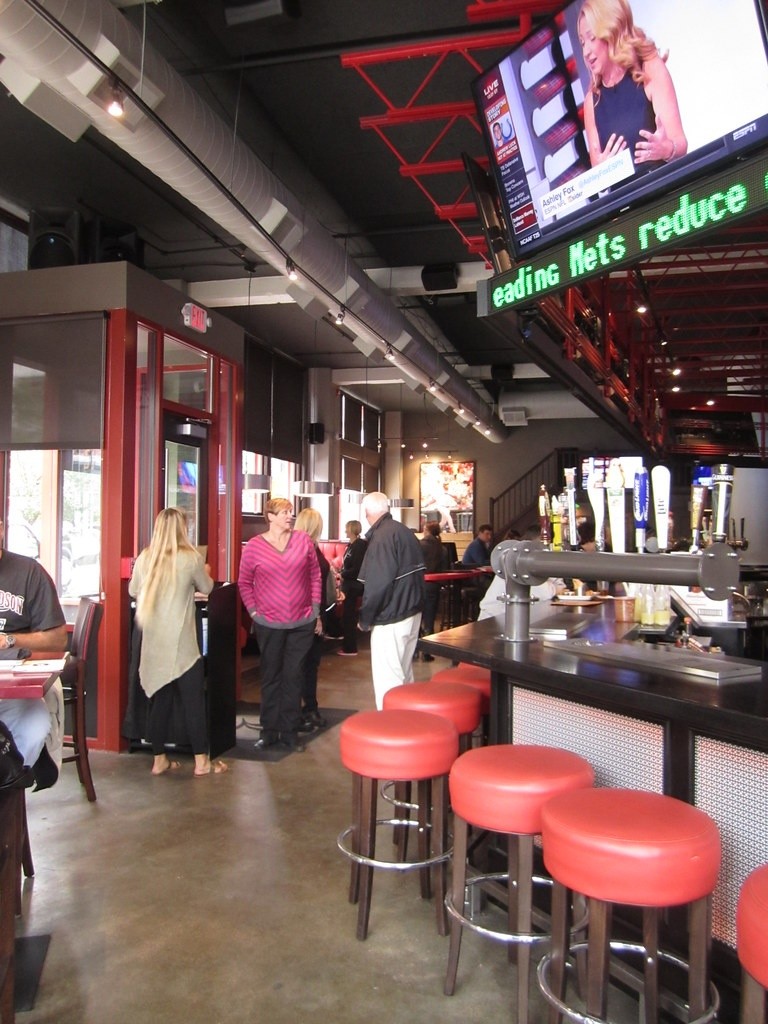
[648,151,651,157]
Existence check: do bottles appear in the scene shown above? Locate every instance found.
[633,584,671,625]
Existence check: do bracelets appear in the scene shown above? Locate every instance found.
[665,141,676,163]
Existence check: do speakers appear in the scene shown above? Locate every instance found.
[28,207,145,271]
[491,363,514,380]
[421,264,459,292]
[309,422,325,443]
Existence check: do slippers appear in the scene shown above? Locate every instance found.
[193,761,228,778]
[150,759,182,778]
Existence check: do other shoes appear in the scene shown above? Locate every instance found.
[299,718,313,731]
[324,633,344,640]
[308,711,326,727]
[420,653,435,662]
[253,737,278,751]
[337,648,358,655]
[281,733,304,750]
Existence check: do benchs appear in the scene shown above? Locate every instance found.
[314,541,364,620]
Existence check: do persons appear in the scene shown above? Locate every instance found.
[238,498,323,752]
[413,520,448,662]
[357,492,427,711]
[334,521,367,657]
[0,520,68,766]
[293,508,336,725]
[572,0,688,192]
[128,508,229,777]
[493,123,509,149]
[462,524,493,590]
[478,522,622,625]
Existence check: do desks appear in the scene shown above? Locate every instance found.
[422,564,495,634]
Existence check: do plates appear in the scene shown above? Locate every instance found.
[557,595,592,601]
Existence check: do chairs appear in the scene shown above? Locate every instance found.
[56,597,97,802]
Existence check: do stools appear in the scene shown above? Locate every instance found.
[536,786,722,1024]
[438,561,481,632]
[338,664,491,943]
[735,863,768,1024]
[443,744,594,1024]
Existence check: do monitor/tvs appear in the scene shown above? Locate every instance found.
[461,0,768,278]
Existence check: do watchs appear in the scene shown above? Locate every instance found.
[4,633,15,648]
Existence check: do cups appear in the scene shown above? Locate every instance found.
[613,596,635,623]
[596,580,609,596]
